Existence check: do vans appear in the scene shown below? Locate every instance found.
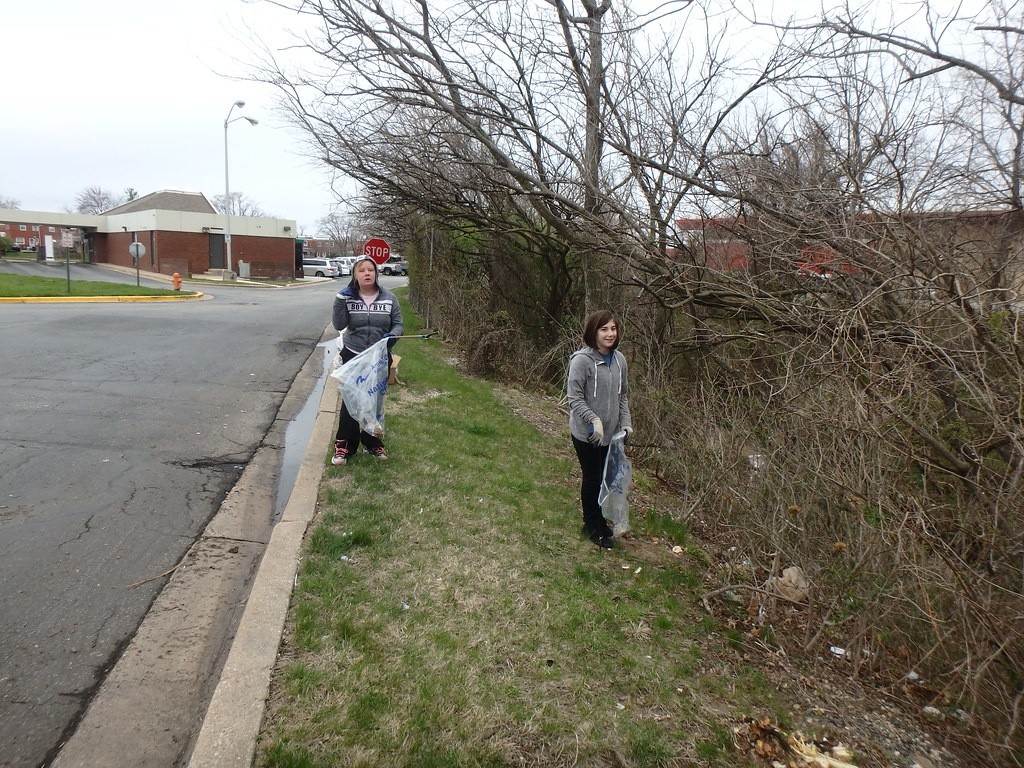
[330,259,352,275]
[337,257,357,272]
[303,258,340,277]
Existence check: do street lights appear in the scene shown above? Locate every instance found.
[224,101,258,270]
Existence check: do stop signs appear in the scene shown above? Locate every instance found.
[362,238,391,264]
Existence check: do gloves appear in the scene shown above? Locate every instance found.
[382,333,396,350]
[587,418,604,448]
[620,425,634,445]
[339,287,359,300]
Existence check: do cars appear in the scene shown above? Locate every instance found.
[376,256,409,276]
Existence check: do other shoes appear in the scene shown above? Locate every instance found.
[594,520,613,537]
[588,531,613,550]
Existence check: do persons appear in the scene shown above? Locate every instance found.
[567,310,634,549]
[331,254,403,467]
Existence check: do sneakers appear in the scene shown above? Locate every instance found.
[329,439,349,465]
[362,443,387,461]
